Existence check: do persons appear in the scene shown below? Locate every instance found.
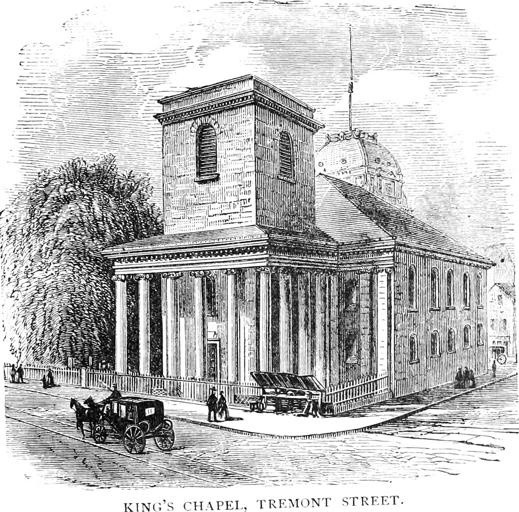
[40,369,55,389]
[10,364,26,383]
[107,383,122,398]
[492,360,496,377]
[455,366,475,388]
[206,387,227,422]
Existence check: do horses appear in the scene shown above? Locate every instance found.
[69,394,101,440]
